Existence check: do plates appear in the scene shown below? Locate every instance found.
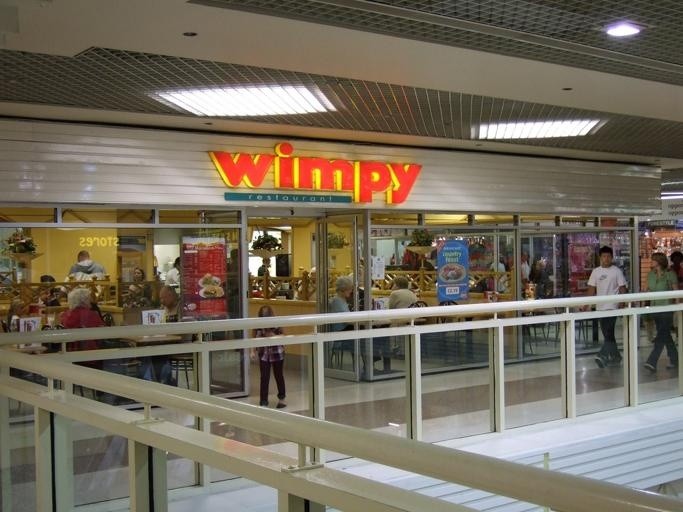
[198,276,224,299]
[439,263,466,282]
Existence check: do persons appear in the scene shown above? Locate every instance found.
[346,273,365,312]
[639,252,679,372]
[6,248,239,399]
[389,249,434,281]
[386,276,417,351]
[327,275,379,375]
[641,299,657,343]
[489,251,551,300]
[248,306,288,409]
[257,258,276,286]
[294,266,316,301]
[666,251,682,348]
[580,245,628,367]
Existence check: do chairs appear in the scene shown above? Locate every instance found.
[330,296,590,371]
[0,313,194,401]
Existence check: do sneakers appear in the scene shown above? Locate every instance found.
[597,356,622,368]
[645,362,656,370]
[260,400,268,406]
[276,400,286,408]
[666,363,678,368]
